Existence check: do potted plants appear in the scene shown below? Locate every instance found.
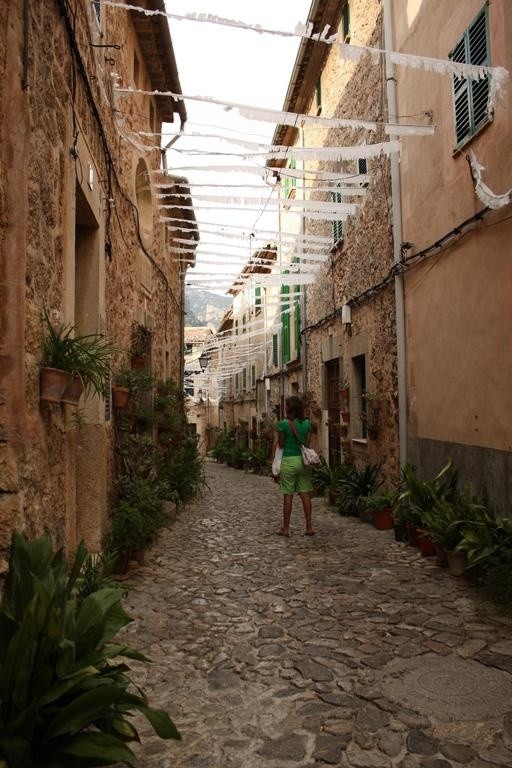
[39,317,117,408]
[112,366,132,408]
[310,450,511,605]
[116,426,205,518]
[131,320,152,370]
[107,519,146,573]
[2,528,188,768]
[211,426,275,482]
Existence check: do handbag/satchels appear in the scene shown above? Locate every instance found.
[301,445,320,467]
[272,443,284,477]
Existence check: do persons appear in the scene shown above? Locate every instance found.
[275,396,318,537]
